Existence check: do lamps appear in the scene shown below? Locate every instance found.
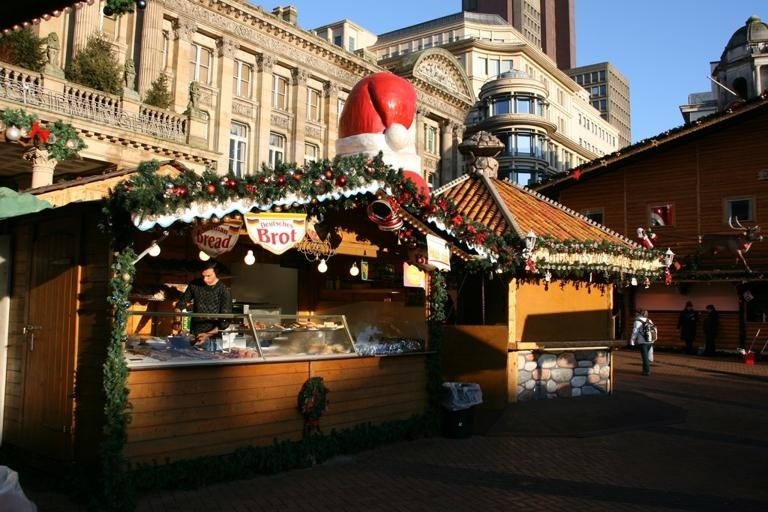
[148,232,161,257]
[6,123,20,140]
[199,250,210,261]
[350,256,359,276]
[244,244,256,266]
[317,253,328,273]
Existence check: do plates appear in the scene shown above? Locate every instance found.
[317,325,336,330]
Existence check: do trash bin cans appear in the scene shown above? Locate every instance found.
[440,381,481,439]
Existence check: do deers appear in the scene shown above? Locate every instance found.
[695,215,764,273]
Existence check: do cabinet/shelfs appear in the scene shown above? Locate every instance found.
[123,311,358,371]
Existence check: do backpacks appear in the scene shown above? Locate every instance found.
[634,318,658,341]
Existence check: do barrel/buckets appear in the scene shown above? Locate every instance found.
[743,351,756,365]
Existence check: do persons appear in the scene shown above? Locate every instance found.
[176,260,233,345]
[677,301,700,354]
[630,307,654,376]
[702,305,720,357]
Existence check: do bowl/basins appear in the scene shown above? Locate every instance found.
[144,339,167,351]
[167,336,191,349]
